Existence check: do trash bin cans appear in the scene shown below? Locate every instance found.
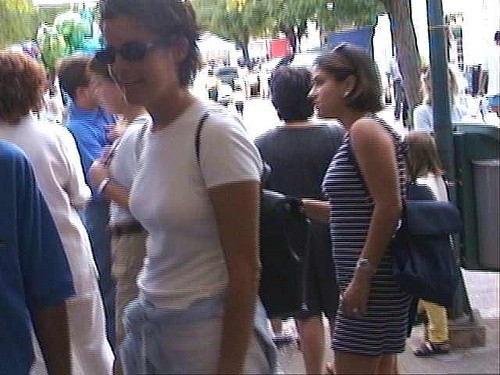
[430,122,500,272]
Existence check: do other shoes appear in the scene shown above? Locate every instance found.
[413,339,450,356]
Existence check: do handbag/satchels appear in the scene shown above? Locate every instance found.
[346,117,462,295]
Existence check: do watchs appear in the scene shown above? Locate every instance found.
[97,175,120,195]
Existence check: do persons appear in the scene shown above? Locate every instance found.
[94,0,279,375]
[404,131,455,357]
[385,58,410,120]
[445,15,465,72]
[412,63,477,139]
[259,42,416,375]
[204,54,271,118]
[254,67,346,375]
[0,42,152,375]
[477,30,500,119]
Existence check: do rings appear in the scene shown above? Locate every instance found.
[352,307,359,313]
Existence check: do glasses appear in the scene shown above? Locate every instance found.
[95,40,168,65]
[332,42,352,63]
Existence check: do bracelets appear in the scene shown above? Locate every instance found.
[357,257,377,274]
[277,194,309,222]
